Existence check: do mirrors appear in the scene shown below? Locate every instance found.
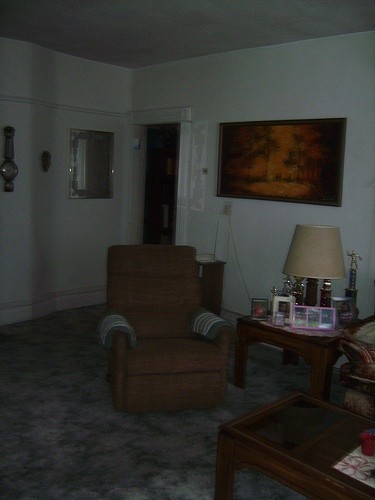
[70,128,114,198]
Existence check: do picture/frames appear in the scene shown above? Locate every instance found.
[273,295,295,325]
[217,118,346,207]
[331,297,357,328]
[293,305,336,331]
[252,298,268,320]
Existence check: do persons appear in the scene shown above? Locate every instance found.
[279,301,289,318]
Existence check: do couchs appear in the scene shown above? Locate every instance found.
[98,244,236,412]
[336,317,375,420]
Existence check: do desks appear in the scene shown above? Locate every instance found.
[197,261,226,318]
[234,314,363,402]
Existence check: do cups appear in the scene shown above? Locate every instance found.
[359,432,375,456]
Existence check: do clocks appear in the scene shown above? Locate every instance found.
[1,127,18,192]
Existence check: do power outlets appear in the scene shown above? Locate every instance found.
[223,201,231,214]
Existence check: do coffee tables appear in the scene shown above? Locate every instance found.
[213,391,375,500]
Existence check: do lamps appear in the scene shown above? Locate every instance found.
[282,223,346,307]
